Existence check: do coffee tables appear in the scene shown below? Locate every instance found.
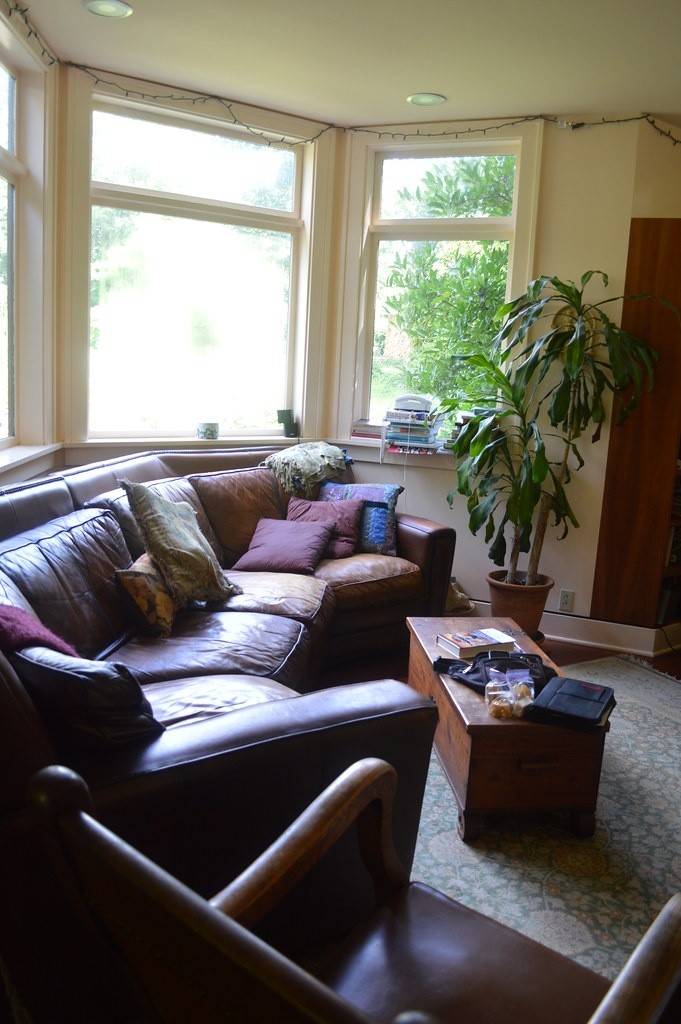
[407,616,611,841]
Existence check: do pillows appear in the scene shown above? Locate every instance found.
[114,553,185,639]
[320,479,404,557]
[286,495,364,558]
[118,478,244,605]
[231,517,339,574]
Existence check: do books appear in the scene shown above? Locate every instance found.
[444,407,501,449]
[436,628,516,658]
[351,418,383,438]
[382,411,445,455]
[527,676,617,730]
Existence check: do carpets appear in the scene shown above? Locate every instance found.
[409,652,680,982]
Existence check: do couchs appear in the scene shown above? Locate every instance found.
[0,444,459,1024]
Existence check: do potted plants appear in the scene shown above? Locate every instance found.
[424,270,680,641]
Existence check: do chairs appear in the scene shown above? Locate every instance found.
[30,759,681,1024]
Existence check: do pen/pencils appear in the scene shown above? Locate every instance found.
[514,641,527,654]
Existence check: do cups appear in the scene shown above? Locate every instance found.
[195,422,219,440]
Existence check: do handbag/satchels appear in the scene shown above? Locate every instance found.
[434,651,558,693]
[532,677,616,726]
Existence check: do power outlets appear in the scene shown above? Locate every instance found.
[558,589,574,611]
